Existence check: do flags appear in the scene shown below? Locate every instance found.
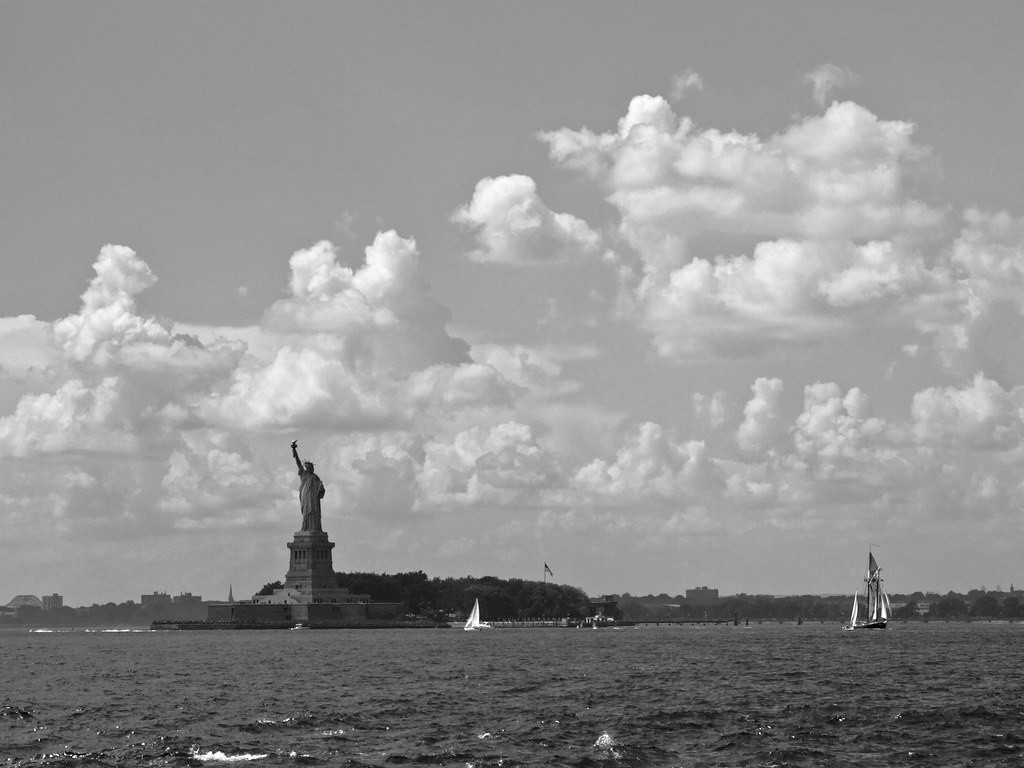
[545,564,553,577]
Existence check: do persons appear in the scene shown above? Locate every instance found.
[291,439,325,532]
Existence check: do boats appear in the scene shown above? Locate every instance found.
[287,623,311,630]
[842,625,855,631]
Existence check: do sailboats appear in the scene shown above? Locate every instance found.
[464,596,492,631]
[851,550,895,628]
